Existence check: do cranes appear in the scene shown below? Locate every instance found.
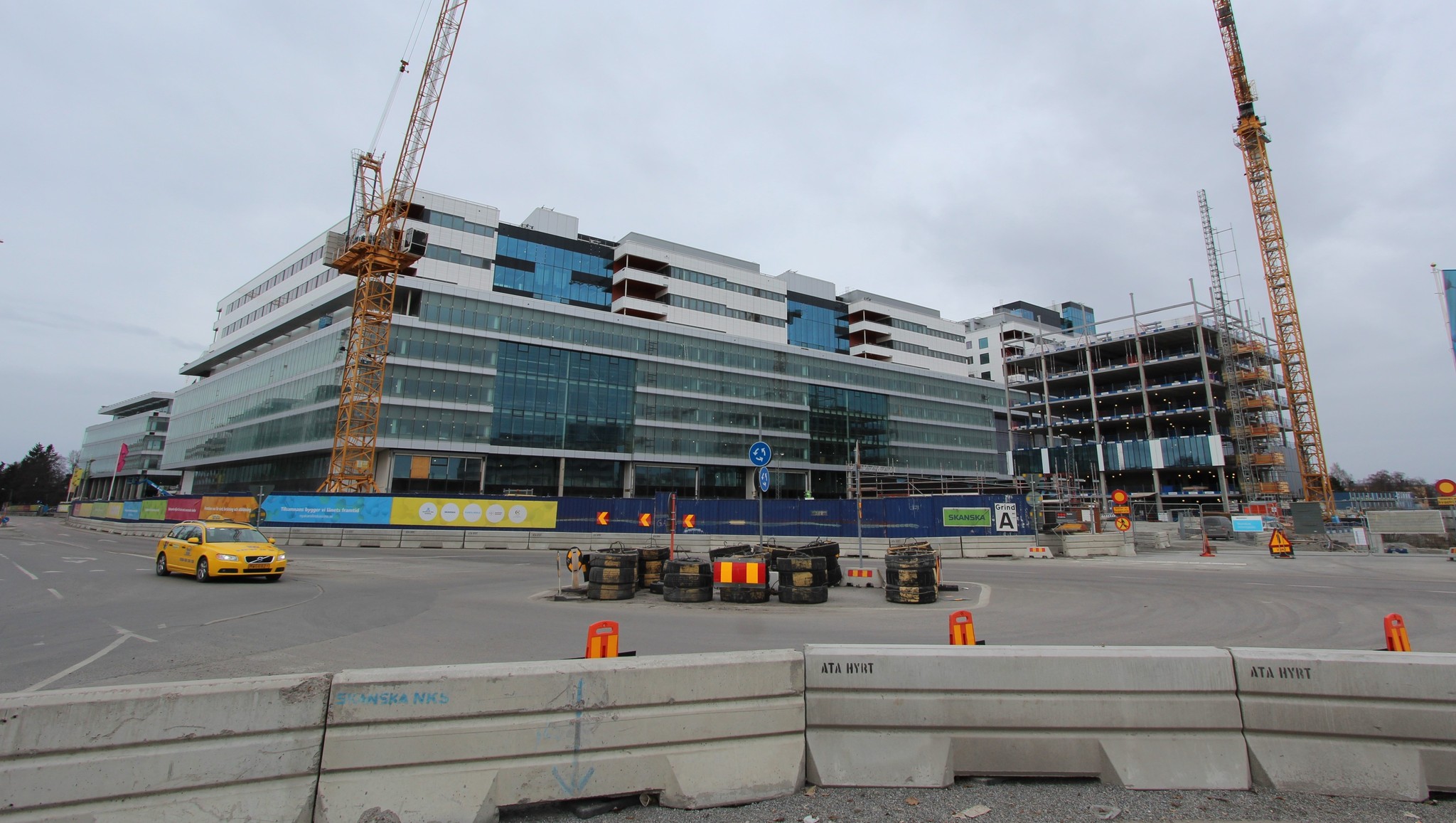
[314,0,468,494]
[1213,0,1337,525]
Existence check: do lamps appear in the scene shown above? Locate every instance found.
[339,346,348,351]
[367,354,375,359]
[387,350,395,355]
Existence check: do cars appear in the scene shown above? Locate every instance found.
[155,514,287,583]
